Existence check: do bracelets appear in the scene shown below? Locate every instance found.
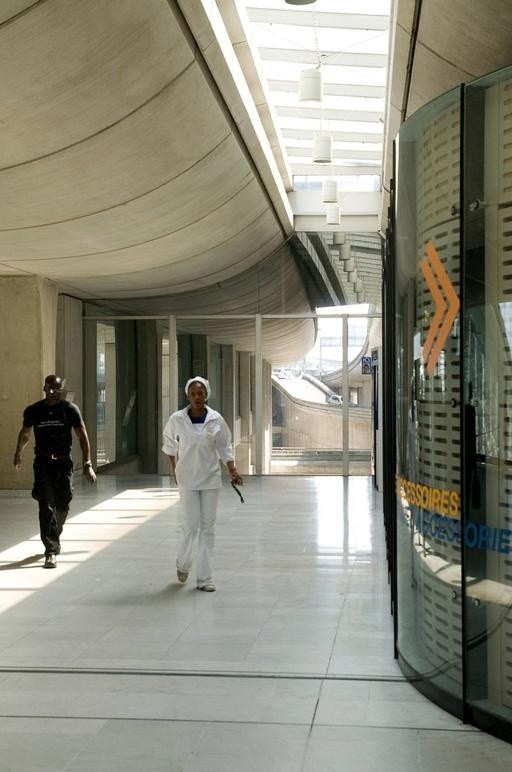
[229,467,237,472]
[83,460,92,465]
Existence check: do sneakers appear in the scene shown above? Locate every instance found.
[177,570,216,591]
[45,555,56,567]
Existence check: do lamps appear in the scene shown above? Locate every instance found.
[297,65,367,304]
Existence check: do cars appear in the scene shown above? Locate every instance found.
[327,395,343,405]
[272,368,292,379]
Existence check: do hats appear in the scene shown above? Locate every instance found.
[184,376,210,401]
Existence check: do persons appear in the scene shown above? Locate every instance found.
[13,373,100,569]
[159,375,245,593]
